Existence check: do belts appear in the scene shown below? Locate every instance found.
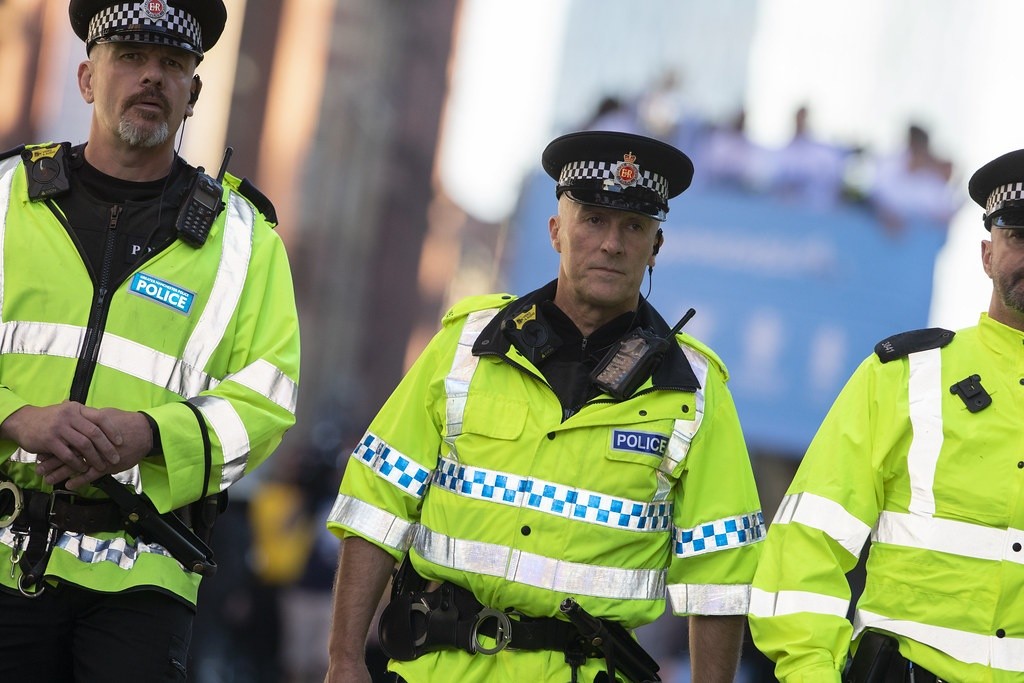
[889,656,949,683]
[421,580,605,659]
[0,482,192,532]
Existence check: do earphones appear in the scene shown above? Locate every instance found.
[188,91,198,105]
[654,243,660,255]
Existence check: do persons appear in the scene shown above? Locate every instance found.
[748,150,1024,683]
[323,132,763,683]
[587,75,953,212]
[0,0,300,683]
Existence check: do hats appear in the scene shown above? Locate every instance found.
[542,131,694,222]
[69,0,227,67]
[969,149,1024,232]
